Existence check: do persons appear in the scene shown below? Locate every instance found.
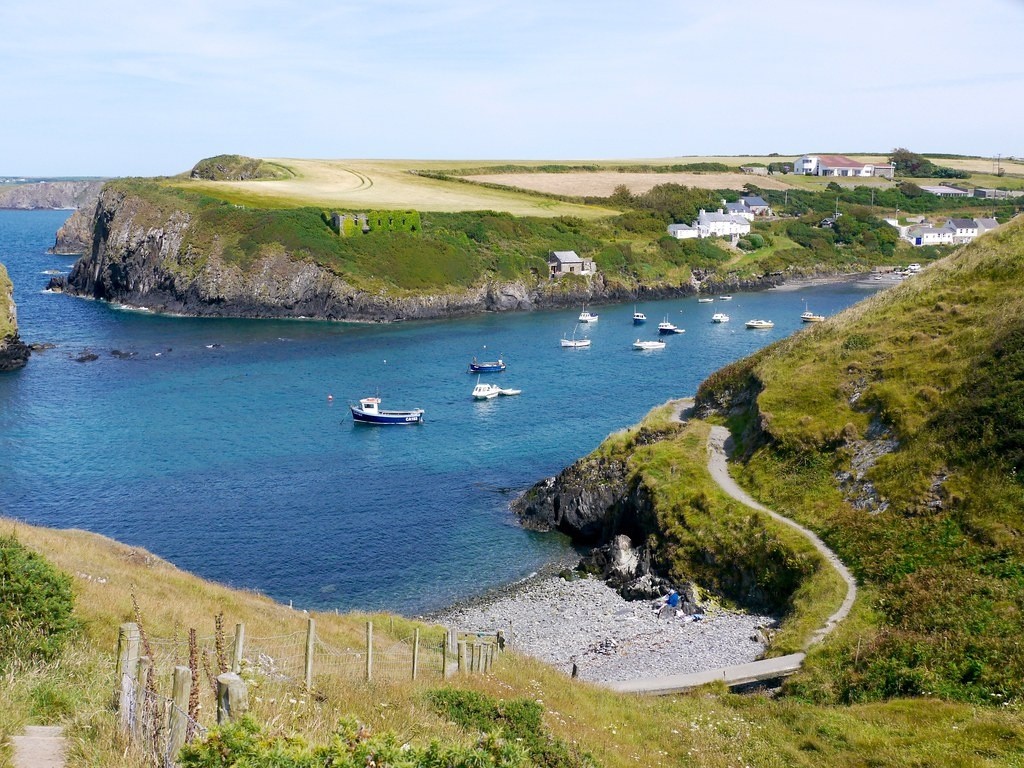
[651,588,679,613]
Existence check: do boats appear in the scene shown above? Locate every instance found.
[631,338,666,350]
[348,386,425,425]
[745,319,775,328]
[719,295,732,300]
[658,313,686,334]
[711,312,730,323]
[578,300,599,322]
[697,298,713,303]
[471,373,523,398]
[633,304,647,323]
[560,333,591,347]
[471,355,506,373]
[800,301,825,322]
